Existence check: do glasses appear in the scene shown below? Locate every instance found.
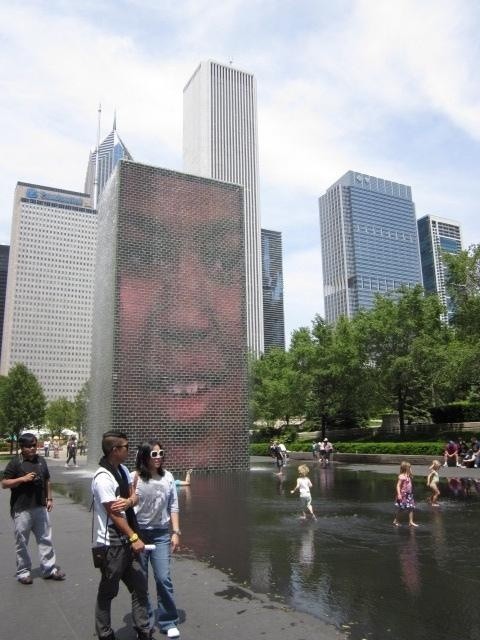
[117,444,130,452]
[23,443,37,448]
[149,450,165,459]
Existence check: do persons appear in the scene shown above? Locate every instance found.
[270,438,333,475]
[176,468,193,486]
[176,486,191,500]
[65,436,79,468]
[442,437,480,469]
[130,440,183,638]
[90,431,155,640]
[53,439,60,458]
[44,437,50,457]
[393,459,421,527]
[118,169,243,477]
[273,476,287,496]
[290,464,318,521]
[445,476,480,497]
[2,433,67,585]
[423,460,442,508]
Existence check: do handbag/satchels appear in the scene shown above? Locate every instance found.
[91,543,108,568]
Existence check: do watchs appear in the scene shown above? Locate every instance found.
[171,529,182,536]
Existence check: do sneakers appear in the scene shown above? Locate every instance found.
[44,570,65,579]
[442,463,479,469]
[18,575,34,585]
[166,626,180,638]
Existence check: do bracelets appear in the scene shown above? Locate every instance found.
[129,532,138,542]
[47,498,52,501]
